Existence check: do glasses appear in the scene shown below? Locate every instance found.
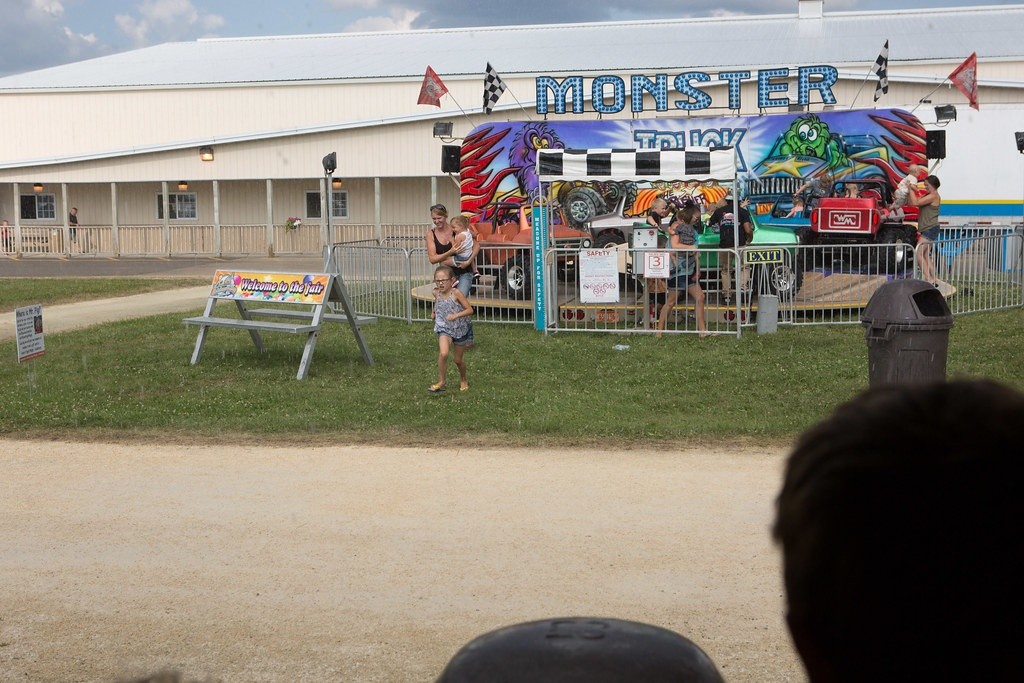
[692,214,701,224]
[429,205,446,213]
[434,278,451,284]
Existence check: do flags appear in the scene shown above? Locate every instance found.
[948,52,979,111]
[417,65,448,106]
[871,41,888,102]
[482,63,506,115]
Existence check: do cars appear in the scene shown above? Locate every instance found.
[632,196,804,307]
[579,191,673,289]
[747,179,927,278]
[466,201,594,297]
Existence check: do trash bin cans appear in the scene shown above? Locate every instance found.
[862,277,954,387]
[756,294,779,334]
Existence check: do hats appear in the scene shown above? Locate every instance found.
[725,194,743,202]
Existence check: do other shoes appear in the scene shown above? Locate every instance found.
[472,270,481,279]
[883,208,890,217]
[699,331,720,338]
[452,279,459,288]
[721,296,731,305]
[740,293,753,306]
[670,266,681,275]
[931,281,940,288]
[692,273,701,281]
[654,333,662,339]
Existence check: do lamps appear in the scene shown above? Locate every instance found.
[178,181,188,191]
[33,184,43,192]
[332,178,342,188]
[200,147,213,161]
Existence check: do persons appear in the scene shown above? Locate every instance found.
[771,374,1023,683]
[793,175,837,197]
[442,216,481,288]
[645,197,750,337]
[428,267,474,392]
[427,203,480,297]
[708,195,753,304]
[781,198,803,219]
[2,220,11,252]
[888,164,920,212]
[907,175,941,283]
[846,184,860,197]
[70,208,80,244]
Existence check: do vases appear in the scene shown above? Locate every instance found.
[290,225,296,229]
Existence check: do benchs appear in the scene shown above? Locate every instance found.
[183,317,319,334]
[246,308,378,325]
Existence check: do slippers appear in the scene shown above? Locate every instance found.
[459,386,469,391]
[428,382,447,392]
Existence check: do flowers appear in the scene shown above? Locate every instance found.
[286,217,301,231]
